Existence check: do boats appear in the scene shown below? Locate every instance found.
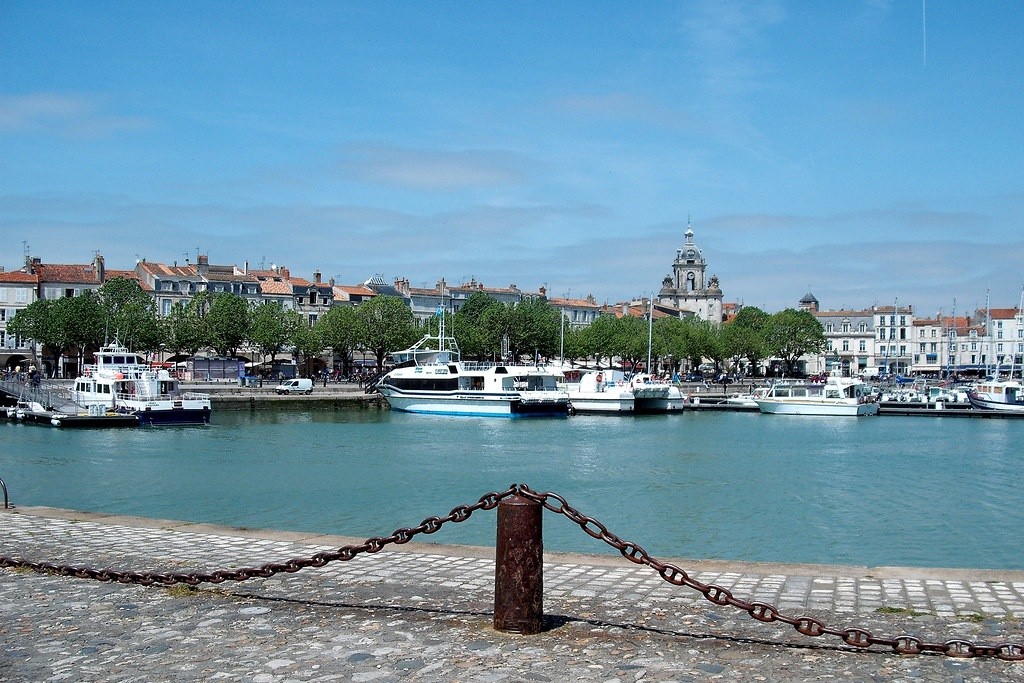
[72,320,213,423]
[726,388,769,405]
[755,376,881,417]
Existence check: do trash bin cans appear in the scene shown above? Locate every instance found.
[237,376,246,387]
[245,376,257,387]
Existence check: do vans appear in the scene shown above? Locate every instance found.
[712,373,735,384]
[275,379,314,396]
[858,368,880,377]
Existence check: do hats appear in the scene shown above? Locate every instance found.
[29,365,36,371]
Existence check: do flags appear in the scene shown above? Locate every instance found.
[436,307,442,317]
[672,374,678,383]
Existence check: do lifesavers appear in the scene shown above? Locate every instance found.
[856,389,861,397]
[618,380,623,387]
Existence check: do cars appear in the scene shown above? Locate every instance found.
[687,373,704,382]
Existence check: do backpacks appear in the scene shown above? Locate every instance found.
[34,372,40,383]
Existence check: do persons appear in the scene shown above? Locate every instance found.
[7,363,40,391]
[887,372,967,384]
[248,367,376,389]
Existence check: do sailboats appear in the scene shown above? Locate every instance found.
[890,284,1024,411]
[376,282,686,415]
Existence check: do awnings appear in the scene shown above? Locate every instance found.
[942,365,1022,372]
